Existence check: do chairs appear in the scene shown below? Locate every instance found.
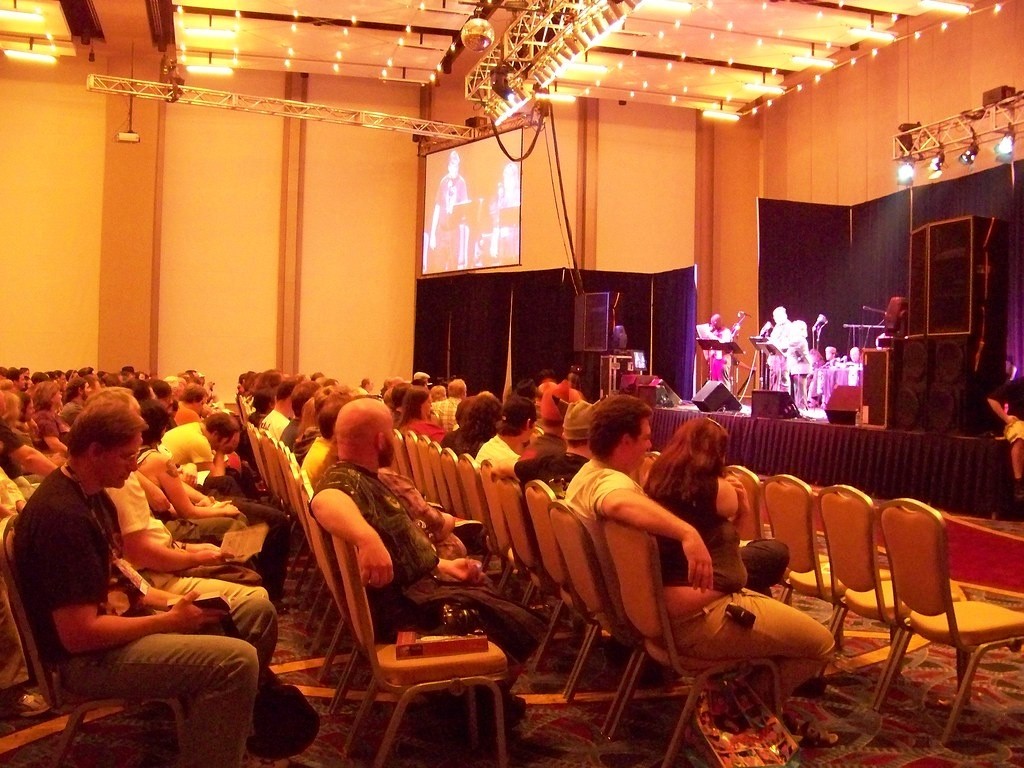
[0,395,1024,768]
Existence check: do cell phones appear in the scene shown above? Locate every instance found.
[726,601,756,627]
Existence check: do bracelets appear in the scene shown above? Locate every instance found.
[182,543,187,550]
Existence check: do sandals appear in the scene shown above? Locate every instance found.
[782,712,839,748]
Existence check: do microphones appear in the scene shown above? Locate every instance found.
[812,314,826,330]
[738,311,741,318]
[841,355,848,362]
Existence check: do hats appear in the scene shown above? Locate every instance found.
[198,373,205,377]
[502,397,536,426]
[414,372,430,379]
[825,346,837,354]
[552,394,606,440]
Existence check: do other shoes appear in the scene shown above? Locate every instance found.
[0,685,50,717]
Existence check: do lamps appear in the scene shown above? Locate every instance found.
[897,156,916,179]
[185,27,236,39]
[959,138,980,165]
[3,48,58,63]
[484,0,646,125]
[792,54,839,68]
[995,122,1016,155]
[701,109,744,121]
[848,28,900,42]
[186,65,232,75]
[0,9,46,23]
[922,0,974,15]
[928,148,945,171]
[744,83,787,94]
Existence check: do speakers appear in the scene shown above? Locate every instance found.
[750,389,801,419]
[824,385,861,426]
[860,215,1009,431]
[574,291,742,413]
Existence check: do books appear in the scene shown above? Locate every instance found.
[219,525,268,563]
[166,591,233,612]
[179,470,211,486]
[396,625,489,661]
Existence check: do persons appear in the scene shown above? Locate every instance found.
[0,365,289,768]
[239,370,835,736]
[988,357,1024,501]
[702,314,732,392]
[765,306,886,417]
[424,149,519,270]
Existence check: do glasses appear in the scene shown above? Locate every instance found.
[120,450,140,460]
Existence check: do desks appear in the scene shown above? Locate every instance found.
[793,364,863,409]
